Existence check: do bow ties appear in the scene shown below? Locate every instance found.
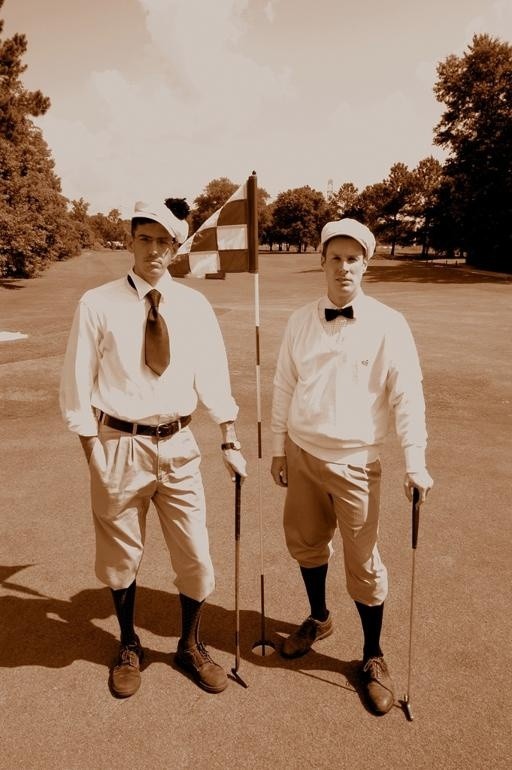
[325,306,353,322]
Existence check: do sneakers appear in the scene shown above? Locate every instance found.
[109,634,143,697]
[175,638,229,693]
[280,610,332,659]
[361,648,395,715]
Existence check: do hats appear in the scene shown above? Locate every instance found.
[321,218,376,261]
[133,199,184,238]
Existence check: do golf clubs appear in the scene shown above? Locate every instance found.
[232,469,247,690]
[403,484,421,723]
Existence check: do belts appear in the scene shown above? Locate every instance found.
[107,414,191,437]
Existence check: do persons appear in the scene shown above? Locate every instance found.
[270,217,434,716]
[58,194,248,698]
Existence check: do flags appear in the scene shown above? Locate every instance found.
[168,180,248,280]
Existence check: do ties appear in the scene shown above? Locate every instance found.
[145,289,171,377]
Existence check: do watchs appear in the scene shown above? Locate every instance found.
[220,441,241,450]
